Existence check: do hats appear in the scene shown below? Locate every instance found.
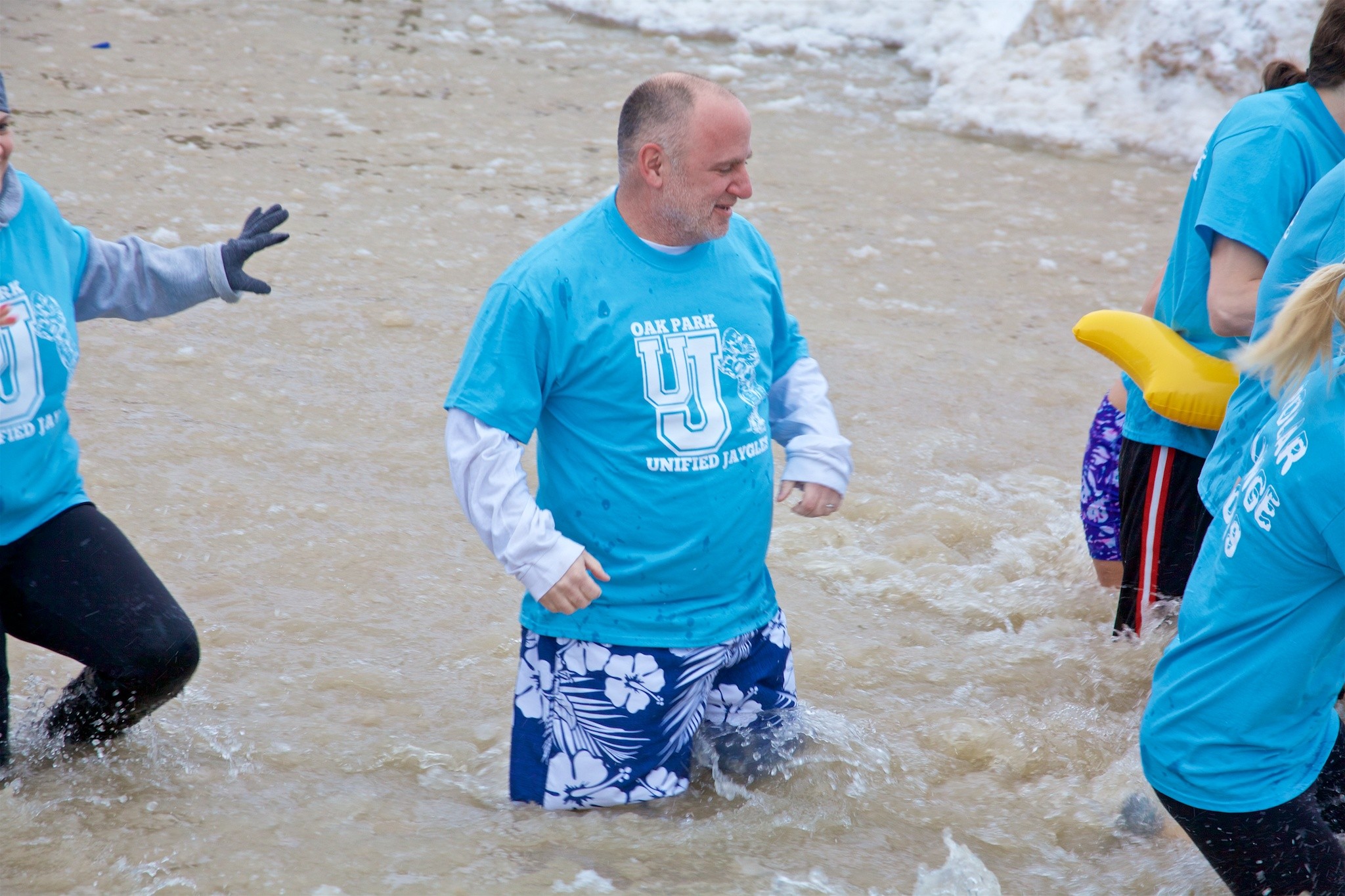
[0,72,9,113]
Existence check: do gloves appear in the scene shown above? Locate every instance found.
[222,204,290,295]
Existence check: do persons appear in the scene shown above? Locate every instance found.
[1194,157,1345,562]
[1082,61,1307,587]
[0,75,291,775]
[1115,0,1345,639]
[444,70,854,811]
[1138,260,1345,895]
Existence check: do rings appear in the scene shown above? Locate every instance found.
[826,504,835,507]
